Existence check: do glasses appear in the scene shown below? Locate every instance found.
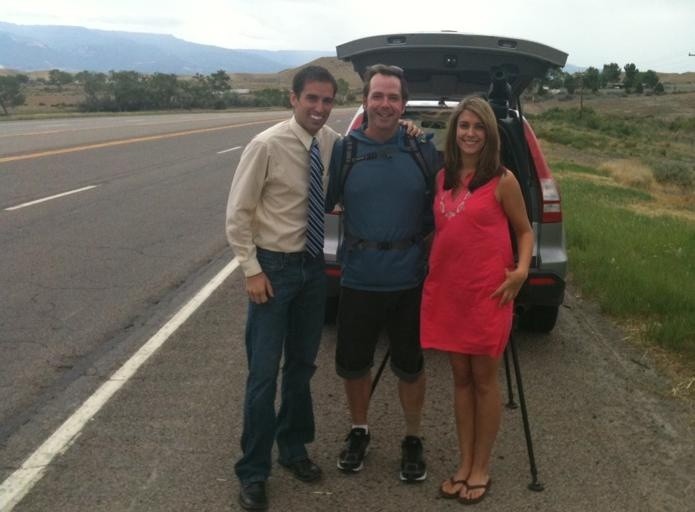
[366,63,405,76]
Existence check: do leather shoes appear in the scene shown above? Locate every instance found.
[236,480,269,511]
[276,452,325,484]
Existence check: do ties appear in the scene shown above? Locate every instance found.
[303,138,326,260]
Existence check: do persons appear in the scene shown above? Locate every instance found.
[322,63,446,482]
[418,95,535,506]
[226,66,424,511]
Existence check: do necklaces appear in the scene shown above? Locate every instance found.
[439,173,472,219]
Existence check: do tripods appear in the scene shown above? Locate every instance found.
[345,303,544,492]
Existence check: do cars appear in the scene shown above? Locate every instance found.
[323,31,567,331]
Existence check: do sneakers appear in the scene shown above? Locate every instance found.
[333,425,378,475]
[395,433,430,485]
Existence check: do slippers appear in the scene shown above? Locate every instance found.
[435,472,466,499]
[456,475,497,506]
